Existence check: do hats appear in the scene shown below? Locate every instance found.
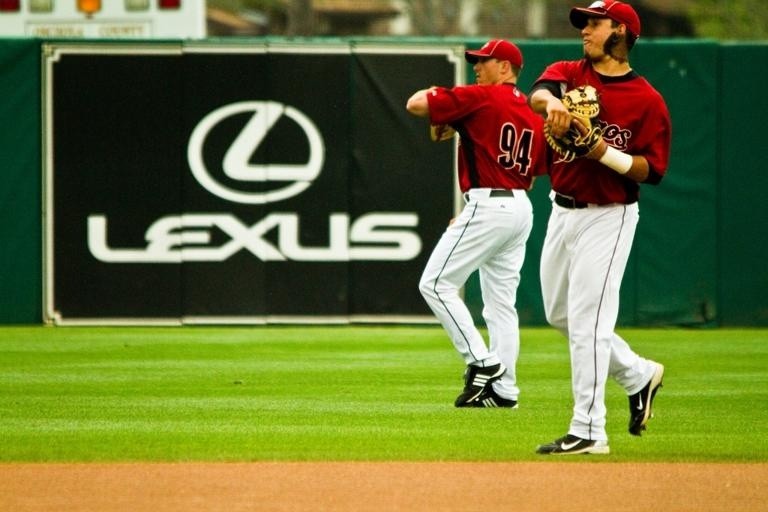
[569,0,641,39]
[465,39,523,69]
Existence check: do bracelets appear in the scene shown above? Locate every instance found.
[598,146,633,174]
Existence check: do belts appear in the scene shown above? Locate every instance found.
[490,190,513,197]
[554,193,587,208]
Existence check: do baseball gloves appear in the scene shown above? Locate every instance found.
[543,83,600,158]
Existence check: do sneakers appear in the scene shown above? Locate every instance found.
[536,434,609,455]
[455,356,519,408]
[629,364,664,435]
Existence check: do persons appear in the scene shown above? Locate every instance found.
[406,39,547,407]
[527,0,671,455]
